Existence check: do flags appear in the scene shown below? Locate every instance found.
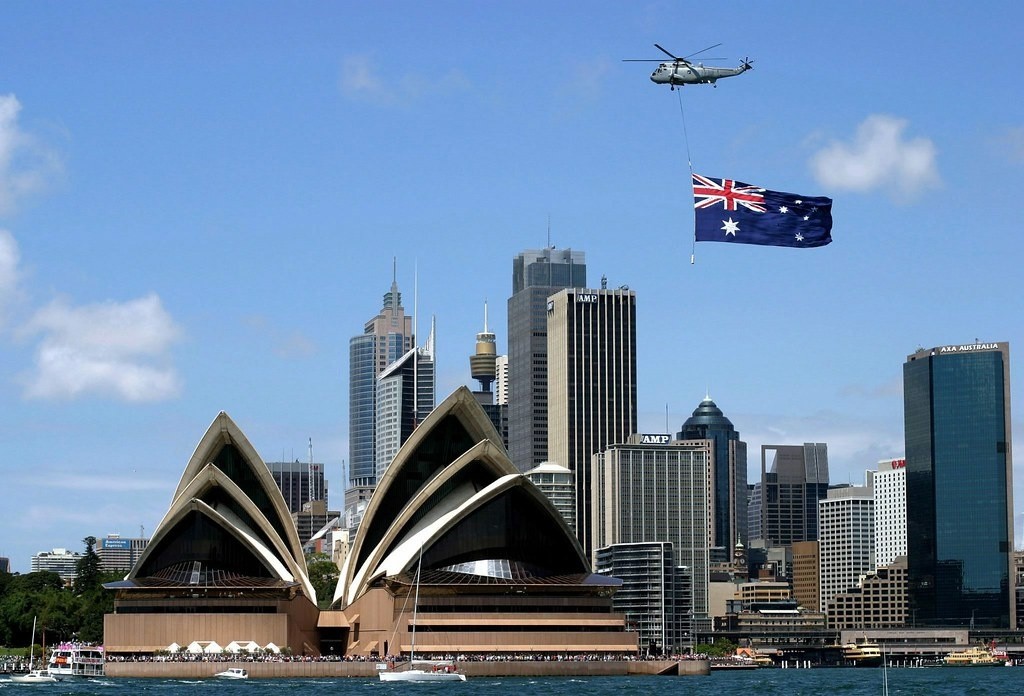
[692,174,833,248]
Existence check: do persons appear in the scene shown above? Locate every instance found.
[413,653,710,662]
[60,638,98,647]
[105,653,410,662]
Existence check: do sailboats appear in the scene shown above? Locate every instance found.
[8,615,62,684]
[378,540,467,684]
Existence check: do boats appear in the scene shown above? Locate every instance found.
[42,641,108,679]
[214,666,249,681]
[940,637,1017,668]
[765,632,883,670]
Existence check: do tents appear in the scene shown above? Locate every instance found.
[166,641,280,653]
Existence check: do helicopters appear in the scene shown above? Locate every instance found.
[621,42,754,92]
